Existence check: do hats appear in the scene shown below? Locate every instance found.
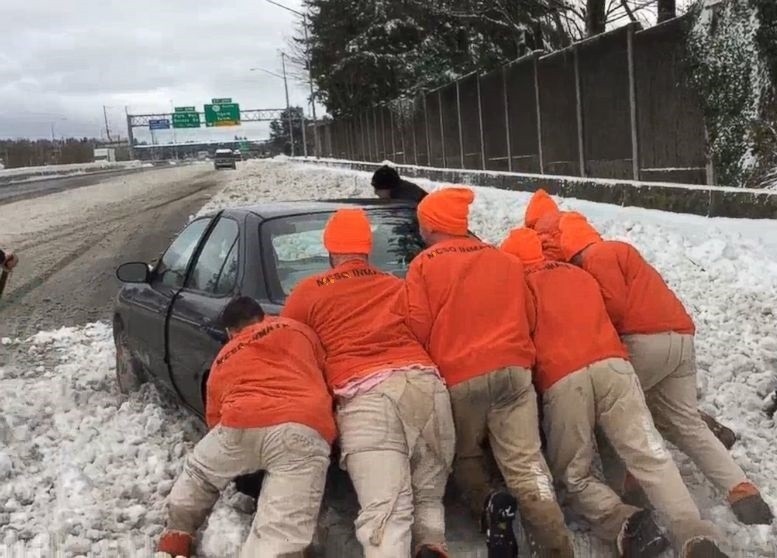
[322,209,373,254]
[525,188,560,227]
[558,211,604,262]
[499,228,545,268]
[370,165,401,189]
[416,186,474,236]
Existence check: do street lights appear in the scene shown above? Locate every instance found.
[250,67,295,156]
[51,117,67,139]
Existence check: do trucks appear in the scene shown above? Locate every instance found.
[93,148,116,162]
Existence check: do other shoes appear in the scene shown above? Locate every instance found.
[479,490,519,558]
[620,473,657,513]
[155,528,194,558]
[415,542,447,558]
[681,537,729,557]
[730,493,775,526]
[699,410,736,450]
[612,509,668,558]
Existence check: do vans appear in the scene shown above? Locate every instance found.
[214,148,236,169]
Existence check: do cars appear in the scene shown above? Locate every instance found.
[240,149,273,159]
[110,198,487,506]
[233,151,242,161]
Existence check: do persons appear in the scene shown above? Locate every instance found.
[153,296,338,558]
[372,165,428,205]
[0,249,18,272]
[280,209,454,558]
[500,188,773,558]
[405,187,573,558]
